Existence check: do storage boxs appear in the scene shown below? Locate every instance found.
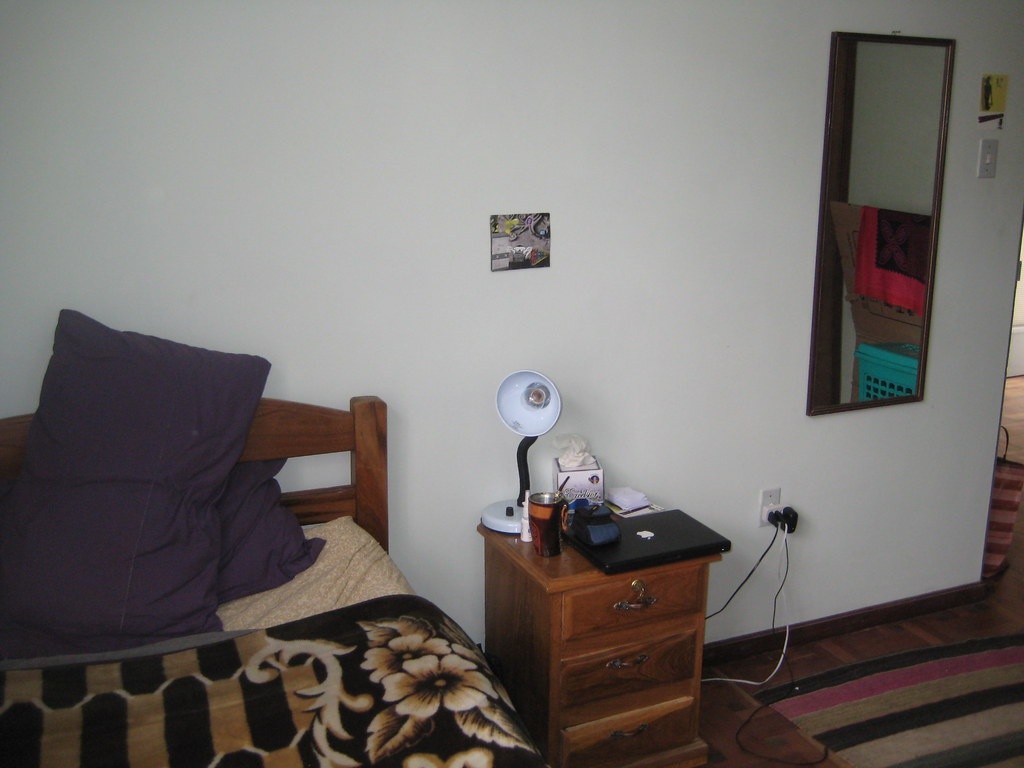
[828,200,932,397]
[854,340,920,402]
[553,457,605,506]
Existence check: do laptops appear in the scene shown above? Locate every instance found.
[560,509,730,574]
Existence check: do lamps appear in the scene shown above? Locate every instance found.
[479,371,563,537]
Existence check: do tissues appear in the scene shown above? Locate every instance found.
[553,434,605,505]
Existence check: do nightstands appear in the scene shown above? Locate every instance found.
[475,497,723,768]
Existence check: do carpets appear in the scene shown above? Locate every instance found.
[753,631,1024,768]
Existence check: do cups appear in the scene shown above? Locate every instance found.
[529,492,569,557]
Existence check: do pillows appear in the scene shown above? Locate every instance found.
[215,458,327,605]
[0,307,271,668]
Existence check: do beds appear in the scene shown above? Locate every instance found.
[0,395,550,768]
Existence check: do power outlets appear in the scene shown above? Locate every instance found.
[757,487,782,529]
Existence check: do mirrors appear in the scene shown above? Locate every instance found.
[804,32,957,415]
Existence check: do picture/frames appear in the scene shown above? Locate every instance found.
[490,212,551,272]
